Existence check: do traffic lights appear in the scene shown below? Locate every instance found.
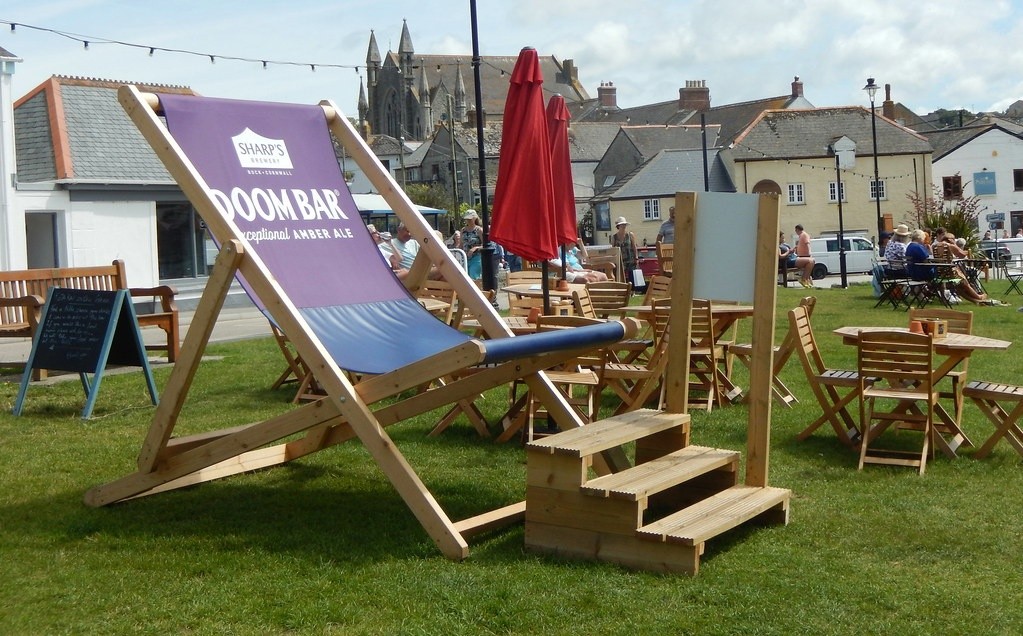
[456,170,463,184]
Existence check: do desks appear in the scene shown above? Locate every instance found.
[498,282,588,316]
[832,326,1012,459]
[955,258,1023,297]
[459,315,608,444]
[618,304,754,400]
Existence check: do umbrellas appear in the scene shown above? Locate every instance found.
[543,94,578,279]
[488,46,558,316]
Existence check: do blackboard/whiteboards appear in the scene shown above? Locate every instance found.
[24,286,152,373]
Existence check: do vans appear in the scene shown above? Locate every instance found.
[799,237,880,280]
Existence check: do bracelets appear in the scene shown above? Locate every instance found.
[636,258,639,260]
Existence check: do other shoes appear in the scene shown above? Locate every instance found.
[799,279,809,288]
[807,281,812,287]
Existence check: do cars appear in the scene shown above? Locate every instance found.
[976,242,1011,267]
[625,247,661,277]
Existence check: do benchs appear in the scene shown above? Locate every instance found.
[0,259,178,381]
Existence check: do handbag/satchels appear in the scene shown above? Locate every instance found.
[633,264,644,286]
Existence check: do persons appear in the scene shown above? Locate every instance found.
[612,217,639,297]
[779,231,816,288]
[367,209,523,307]
[656,206,676,244]
[558,236,608,283]
[794,224,814,286]
[878,225,1023,306]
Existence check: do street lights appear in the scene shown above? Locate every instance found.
[861,76,881,244]
[400,137,405,195]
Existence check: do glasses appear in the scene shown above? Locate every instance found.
[372,231,377,234]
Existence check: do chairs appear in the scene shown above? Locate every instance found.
[264,236,1023,480]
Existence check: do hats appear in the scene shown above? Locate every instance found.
[894,224,911,236]
[463,210,477,220]
[615,217,628,227]
